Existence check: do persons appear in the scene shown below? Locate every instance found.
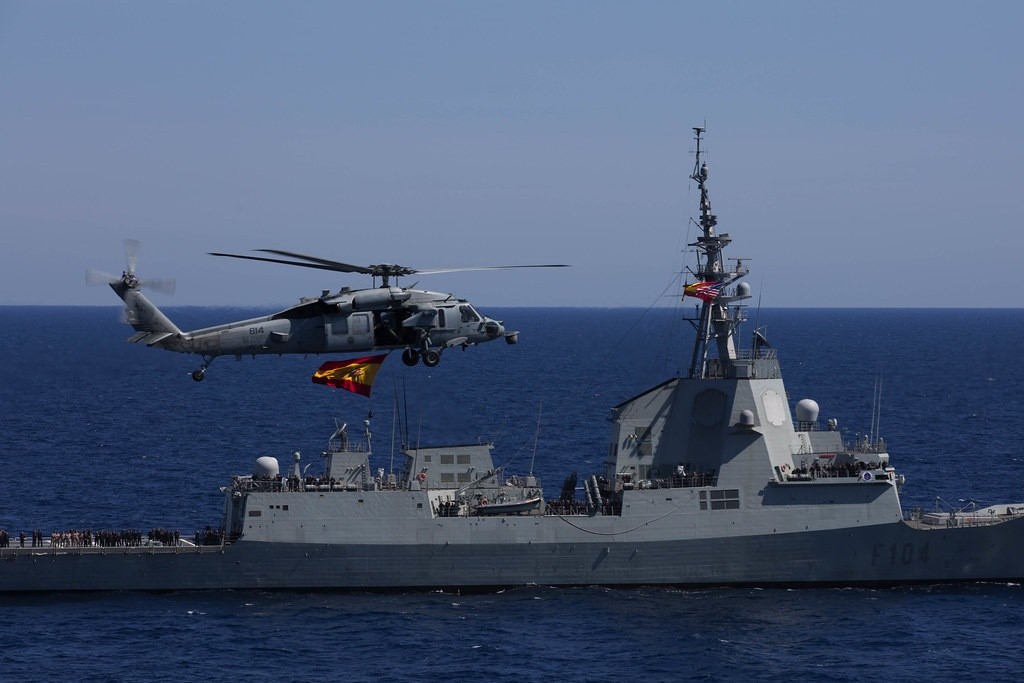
[670,471,713,488]
[252,472,300,492]
[882,460,887,469]
[50,529,142,547]
[19,531,25,547]
[194,530,200,546]
[204,524,225,545]
[305,475,335,486]
[547,499,580,515]
[439,502,460,517]
[32,529,43,547]
[147,528,179,546]
[796,460,870,478]
[0,529,10,548]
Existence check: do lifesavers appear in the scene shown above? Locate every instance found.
[420,473,426,480]
[481,499,489,505]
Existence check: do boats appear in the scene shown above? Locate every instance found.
[474,489,542,514]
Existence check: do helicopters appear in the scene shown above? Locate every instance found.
[86,239,573,382]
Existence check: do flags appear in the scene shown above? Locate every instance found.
[312,353,388,398]
[685,281,722,305]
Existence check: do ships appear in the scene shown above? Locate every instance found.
[0,120,1024,592]
